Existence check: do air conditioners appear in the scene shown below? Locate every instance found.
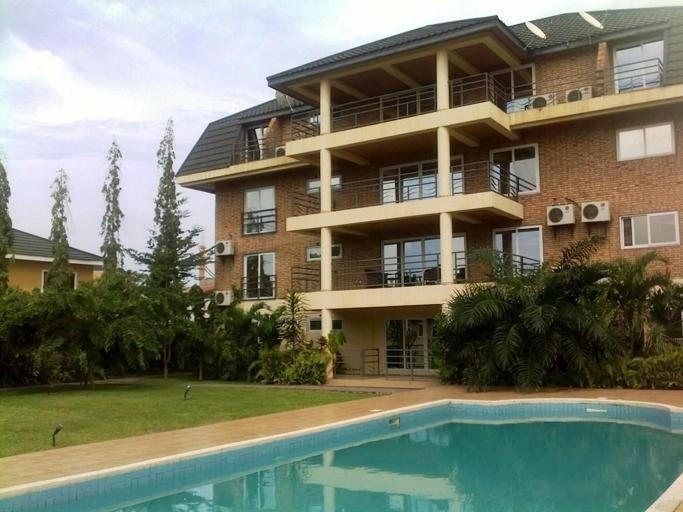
[581,201,610,223]
[529,93,557,108]
[215,290,234,306]
[275,146,285,158]
[547,204,576,226]
[566,86,593,102]
[215,239,234,256]
